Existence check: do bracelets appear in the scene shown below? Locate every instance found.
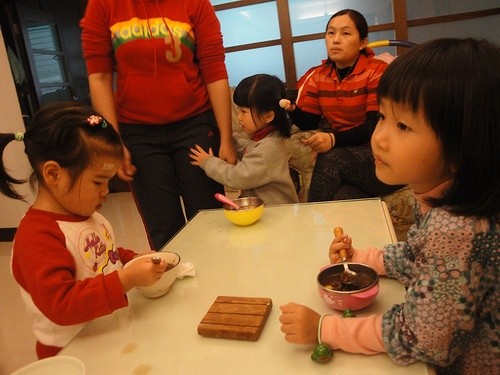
[318,313,333,345]
[328,132,333,147]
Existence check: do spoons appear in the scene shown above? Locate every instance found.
[334,227,356,284]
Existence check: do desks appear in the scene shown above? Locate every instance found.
[55,197,434,375]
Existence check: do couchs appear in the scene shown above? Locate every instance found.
[284,42,420,241]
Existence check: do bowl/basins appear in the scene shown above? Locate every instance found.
[122,251,181,298]
[317,262,380,311]
[10,355,86,375]
[223,197,265,227]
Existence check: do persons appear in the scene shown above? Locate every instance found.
[0,104,167,362]
[279,37,500,375]
[80,0,237,254]
[281,9,408,203]
[189,73,301,206]
